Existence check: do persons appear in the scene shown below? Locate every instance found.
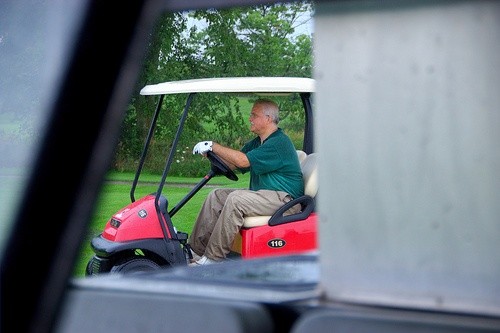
[186,99,304,265]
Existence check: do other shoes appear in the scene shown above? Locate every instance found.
[186,248,225,266]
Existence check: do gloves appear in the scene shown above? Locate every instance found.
[192,141,212,156]
